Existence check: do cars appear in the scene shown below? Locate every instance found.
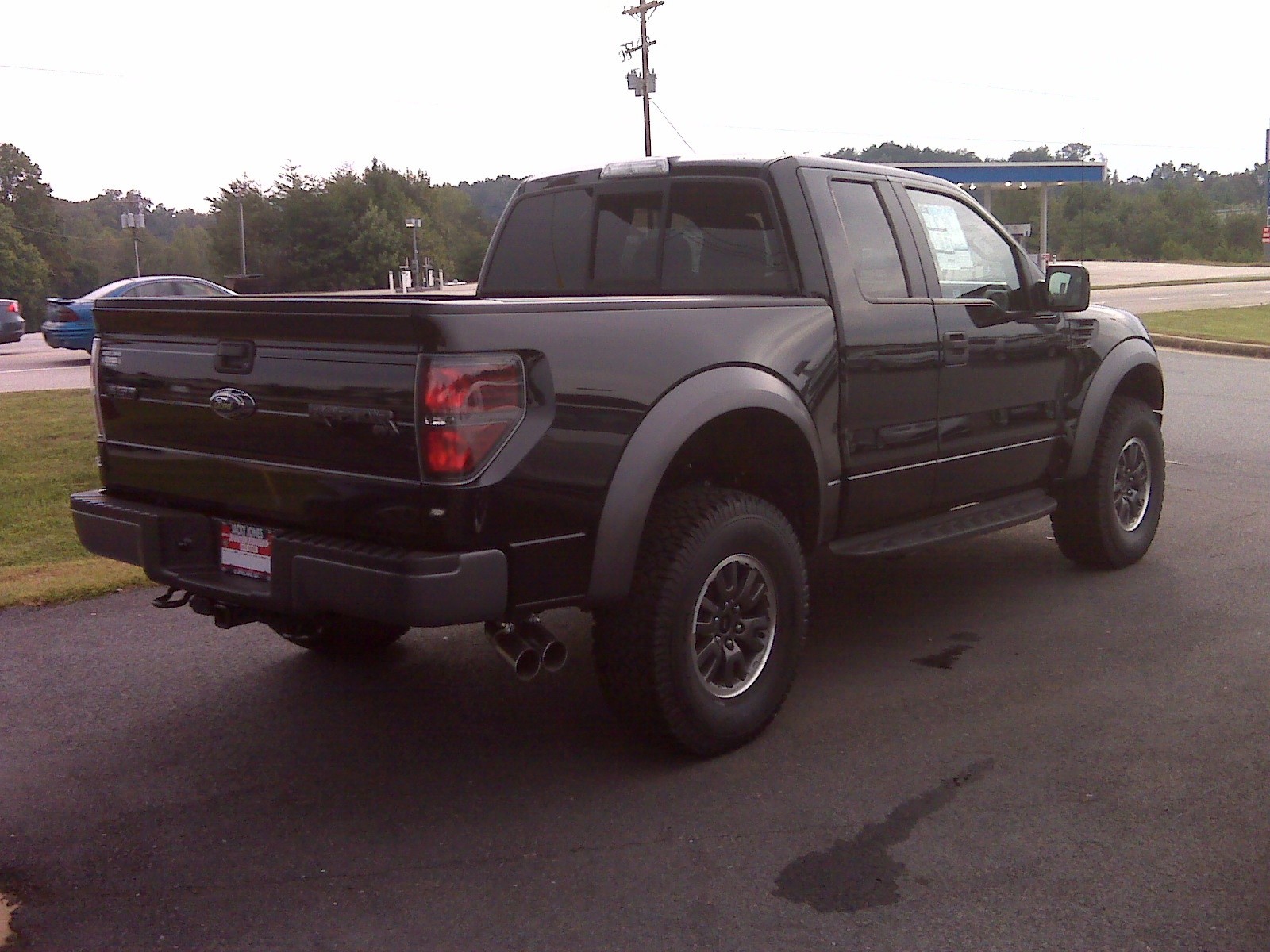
[0,298,25,345]
[39,273,240,360]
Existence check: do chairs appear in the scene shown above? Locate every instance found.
[699,215,767,297]
[629,230,694,296]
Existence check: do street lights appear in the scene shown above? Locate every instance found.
[404,218,423,292]
[120,210,146,277]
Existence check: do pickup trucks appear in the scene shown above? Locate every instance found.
[63,152,1171,765]
